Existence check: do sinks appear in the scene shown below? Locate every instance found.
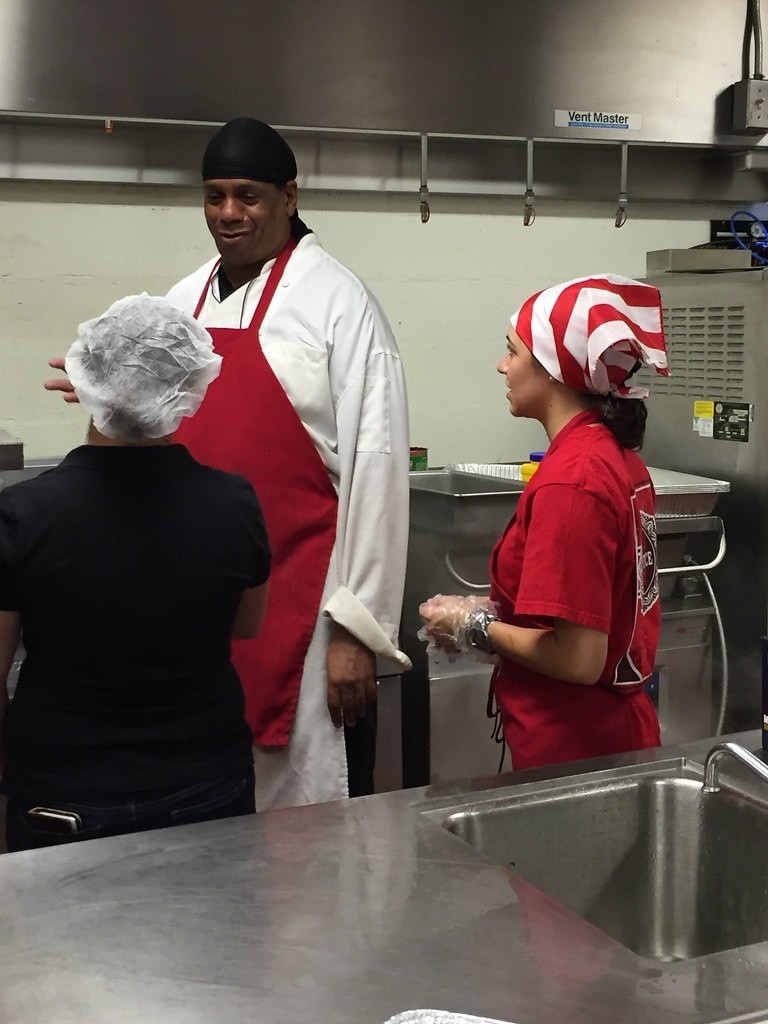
[410,756,768,964]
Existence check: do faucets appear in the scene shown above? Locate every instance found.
[701,741,768,793]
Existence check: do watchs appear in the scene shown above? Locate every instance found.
[466,615,501,655]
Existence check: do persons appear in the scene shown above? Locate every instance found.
[44,117,414,815]
[418,273,661,773]
[1,291,272,851]
